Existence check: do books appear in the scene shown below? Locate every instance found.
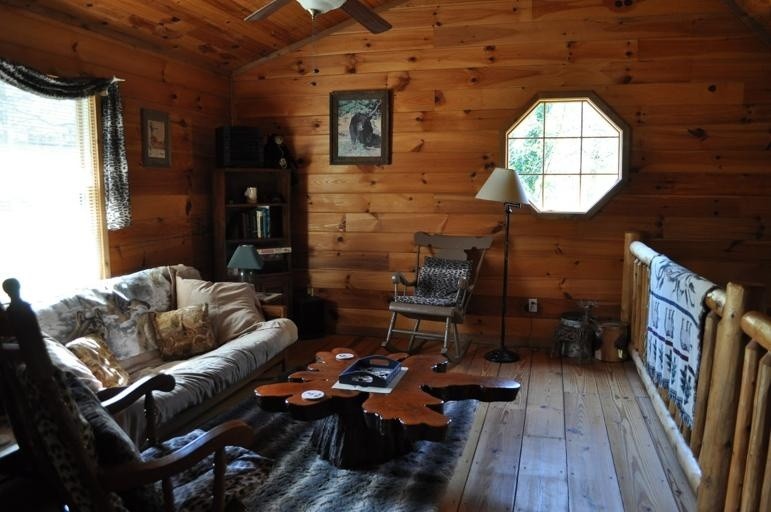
[255,246,292,256]
[239,204,270,239]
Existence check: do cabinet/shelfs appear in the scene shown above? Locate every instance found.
[209,165,296,324]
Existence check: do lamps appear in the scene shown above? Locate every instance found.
[226,243,267,287]
[469,165,537,363]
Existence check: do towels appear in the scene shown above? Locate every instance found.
[646,248,727,433]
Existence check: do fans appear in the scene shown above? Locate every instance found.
[241,0,398,36]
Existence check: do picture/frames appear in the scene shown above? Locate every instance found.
[327,87,392,166]
[139,108,173,169]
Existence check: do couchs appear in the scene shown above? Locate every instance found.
[9,260,300,460]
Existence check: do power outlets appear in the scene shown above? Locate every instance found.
[527,297,537,314]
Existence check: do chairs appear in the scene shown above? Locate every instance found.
[379,229,495,357]
[0,275,257,511]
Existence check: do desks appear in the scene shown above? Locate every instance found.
[249,341,526,473]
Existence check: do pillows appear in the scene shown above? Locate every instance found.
[63,272,264,395]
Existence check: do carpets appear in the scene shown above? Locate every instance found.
[149,358,485,512]
